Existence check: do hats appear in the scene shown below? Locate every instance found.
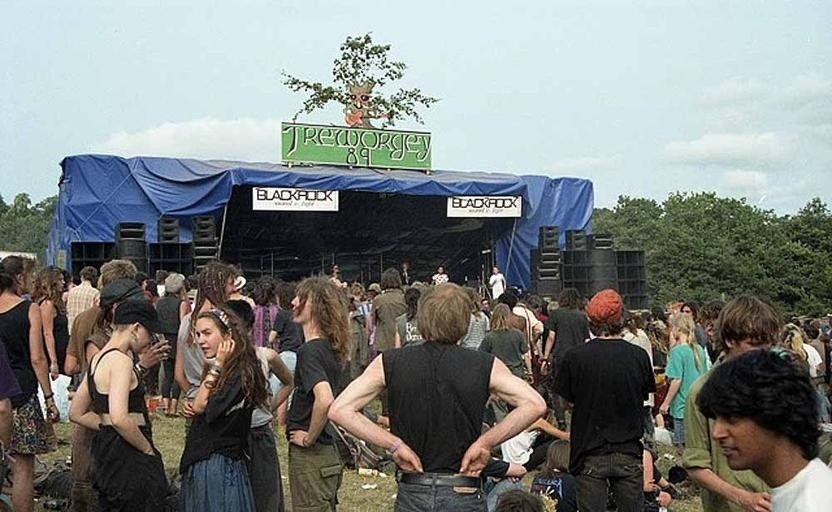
[585,287,624,326]
[163,273,187,293]
[114,298,181,337]
[98,277,146,309]
[232,275,247,292]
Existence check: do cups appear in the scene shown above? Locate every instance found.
[150,333,171,358]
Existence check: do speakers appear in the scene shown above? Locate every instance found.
[115,215,217,271]
[530,226,619,310]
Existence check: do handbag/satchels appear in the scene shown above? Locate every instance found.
[552,363,577,404]
[527,347,551,388]
[324,415,393,473]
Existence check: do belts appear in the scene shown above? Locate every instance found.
[393,469,484,489]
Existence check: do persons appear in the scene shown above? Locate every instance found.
[0,247,832,512]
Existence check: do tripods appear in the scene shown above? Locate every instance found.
[478,265,491,299]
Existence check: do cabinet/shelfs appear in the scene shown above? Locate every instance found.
[529,226,651,311]
[71,214,220,287]
